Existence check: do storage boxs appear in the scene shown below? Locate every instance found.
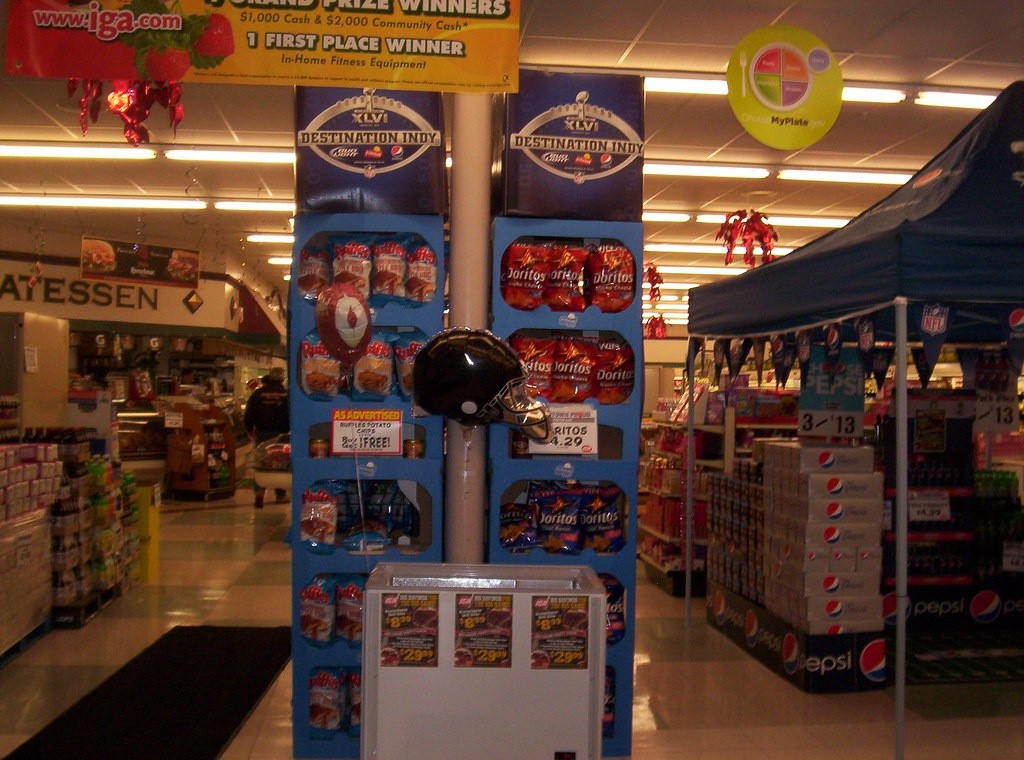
[662,374,886,633]
[137,481,163,538]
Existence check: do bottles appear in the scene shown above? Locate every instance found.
[908,545,963,576]
[63,463,87,495]
[23,428,45,443]
[90,488,121,520]
[974,470,1018,504]
[909,462,960,487]
[694,464,707,495]
[46,427,87,457]
[52,496,91,608]
[657,398,679,424]
[874,414,891,445]
[978,560,1016,580]
[91,553,122,589]
[87,454,121,487]
[644,458,681,494]
[121,470,140,527]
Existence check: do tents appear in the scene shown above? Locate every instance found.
[687,81,1024,757]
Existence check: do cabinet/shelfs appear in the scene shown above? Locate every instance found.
[166,403,237,502]
[488,217,644,760]
[288,211,445,760]
[640,407,875,603]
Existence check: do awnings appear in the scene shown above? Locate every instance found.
[239,282,286,346]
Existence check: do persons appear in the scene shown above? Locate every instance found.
[244,371,290,509]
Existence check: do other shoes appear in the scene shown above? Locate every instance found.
[276,494,290,503]
[254,491,265,507]
